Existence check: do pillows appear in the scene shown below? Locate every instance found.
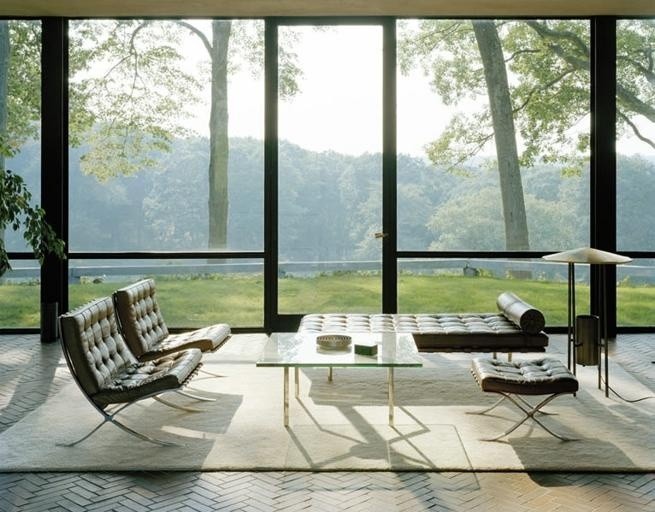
[496,292,546,332]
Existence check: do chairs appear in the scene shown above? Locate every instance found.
[56,297,207,448]
[111,278,233,401]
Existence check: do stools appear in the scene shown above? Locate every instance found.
[463,357,582,443]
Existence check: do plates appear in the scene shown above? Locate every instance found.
[315,333,352,351]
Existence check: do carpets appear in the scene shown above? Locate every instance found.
[0,330,654,472]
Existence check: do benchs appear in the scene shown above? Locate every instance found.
[297,314,548,352]
